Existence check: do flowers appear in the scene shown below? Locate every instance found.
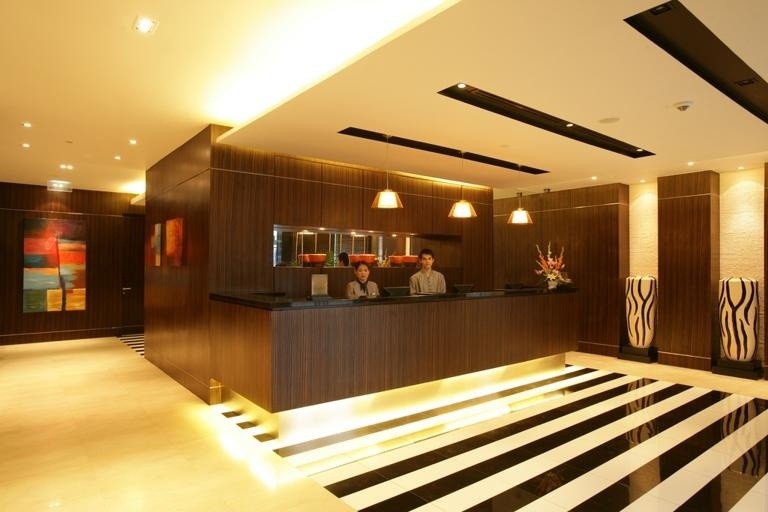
[534,239,572,286]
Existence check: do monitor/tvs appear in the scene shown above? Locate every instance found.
[504,283,523,289]
[382,286,411,297]
[452,283,473,293]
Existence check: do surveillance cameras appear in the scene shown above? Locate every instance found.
[674,102,691,112]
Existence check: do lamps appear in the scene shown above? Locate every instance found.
[371,135,403,208]
[448,150,478,218]
[507,165,533,224]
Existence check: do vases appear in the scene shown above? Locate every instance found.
[547,279,558,290]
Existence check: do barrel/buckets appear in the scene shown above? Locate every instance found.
[298,254,308,267]
[308,253,327,267]
[402,255,418,269]
[388,256,402,267]
[359,253,376,266]
[349,255,359,266]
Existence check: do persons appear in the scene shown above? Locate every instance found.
[336,252,349,266]
[409,248,446,296]
[346,261,379,297]
